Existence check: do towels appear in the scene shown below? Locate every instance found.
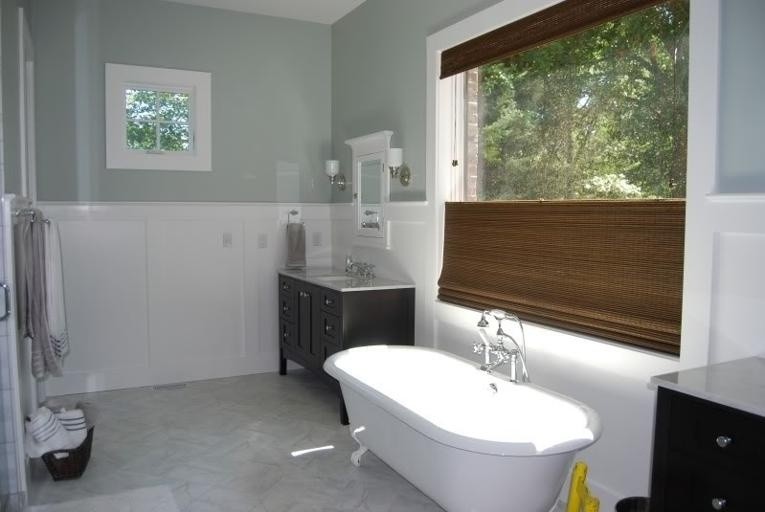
[13,209,71,382]
[24,401,85,458]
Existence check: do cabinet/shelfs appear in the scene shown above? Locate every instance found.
[279,275,343,393]
[647,387,765,510]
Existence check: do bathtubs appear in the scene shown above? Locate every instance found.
[323,338,602,512]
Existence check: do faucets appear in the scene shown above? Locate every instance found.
[342,254,376,280]
[473,327,530,383]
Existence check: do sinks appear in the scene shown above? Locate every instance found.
[313,273,376,289]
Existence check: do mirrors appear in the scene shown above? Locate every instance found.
[343,130,392,250]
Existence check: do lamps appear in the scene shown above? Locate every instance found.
[324,158,347,191]
[385,145,411,188]
